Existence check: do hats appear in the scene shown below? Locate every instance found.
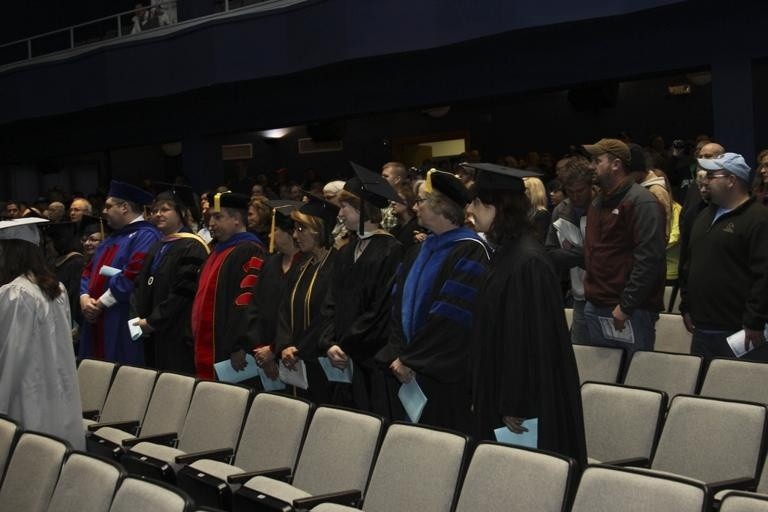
[206,192,251,210]
[581,138,633,164]
[260,189,341,237]
[343,160,406,212]
[697,151,751,181]
[425,160,545,206]
[108,176,195,214]
[1,211,111,249]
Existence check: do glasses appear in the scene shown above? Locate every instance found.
[704,171,731,180]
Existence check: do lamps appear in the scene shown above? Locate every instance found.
[685,71,712,87]
[416,104,451,119]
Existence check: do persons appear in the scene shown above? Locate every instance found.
[2,137,767,473]
